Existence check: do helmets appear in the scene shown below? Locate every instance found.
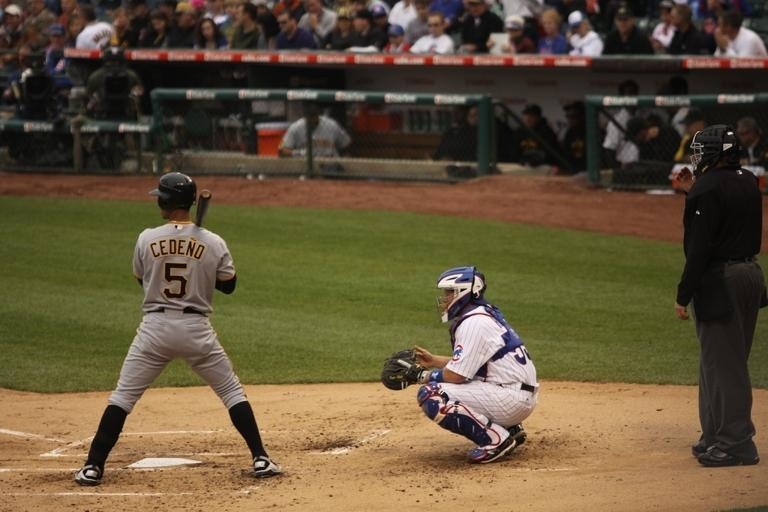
[690,123,742,177]
[435,266,487,322]
[148,171,197,207]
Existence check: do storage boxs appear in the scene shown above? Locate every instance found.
[256,122,292,158]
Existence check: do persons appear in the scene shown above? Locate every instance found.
[74,170,283,486]
[396,267,538,465]
[673,124,765,465]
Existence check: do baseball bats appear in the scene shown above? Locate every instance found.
[196,190,211,226]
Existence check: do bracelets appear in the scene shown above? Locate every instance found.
[430,368,443,383]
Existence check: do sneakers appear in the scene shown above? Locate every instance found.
[73,462,102,485]
[253,456,283,479]
[466,421,517,464]
[697,440,761,467]
[691,432,714,458]
[504,423,528,458]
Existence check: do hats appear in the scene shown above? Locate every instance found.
[369,5,387,17]
[4,4,125,85]
[568,9,587,34]
[387,24,404,38]
[514,97,711,129]
[504,15,526,32]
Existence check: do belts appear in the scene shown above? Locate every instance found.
[499,380,537,394]
[147,307,204,314]
[723,256,759,264]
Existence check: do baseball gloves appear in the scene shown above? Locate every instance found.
[382,350,429,390]
